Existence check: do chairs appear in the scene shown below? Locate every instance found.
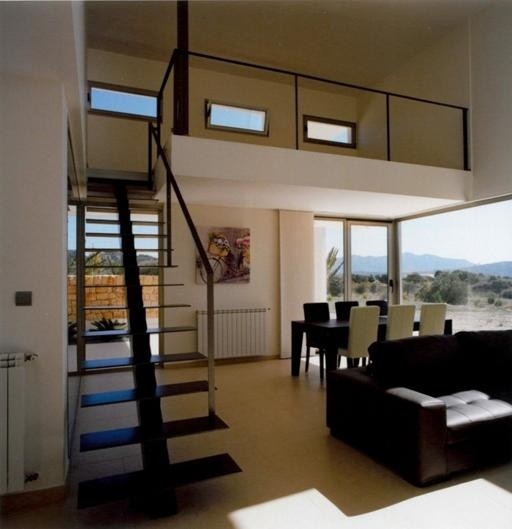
[419,303,447,336]
[337,306,380,369]
[335,301,359,367]
[386,305,415,340]
[366,300,388,317]
[303,303,341,383]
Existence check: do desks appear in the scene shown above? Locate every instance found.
[291,317,452,390]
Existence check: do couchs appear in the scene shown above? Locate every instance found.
[325,329,512,488]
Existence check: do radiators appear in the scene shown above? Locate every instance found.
[196,308,270,359]
[0,352,38,494]
